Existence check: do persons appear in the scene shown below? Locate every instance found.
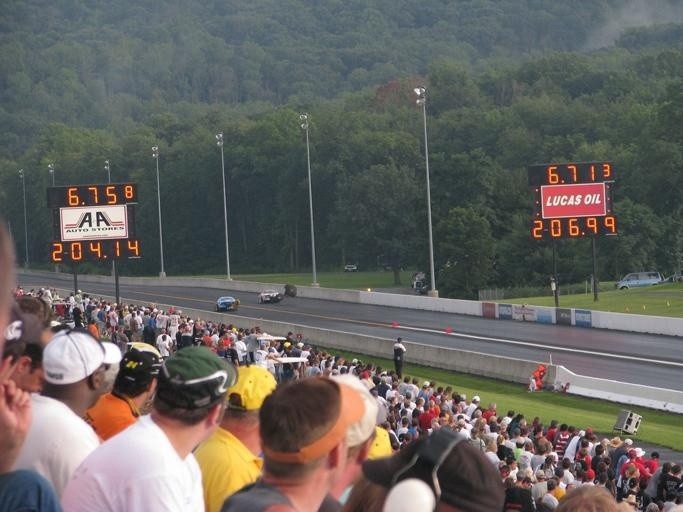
[362,358,683,511]
[393,336,406,379]
[1,284,388,511]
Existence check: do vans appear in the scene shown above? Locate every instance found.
[615,272,662,291]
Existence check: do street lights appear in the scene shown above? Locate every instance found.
[300,112,320,288]
[151,146,167,280]
[18,170,31,271]
[215,132,233,282]
[48,164,60,273]
[413,86,439,298]
[104,159,115,277]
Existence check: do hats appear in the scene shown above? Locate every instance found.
[39,328,124,386]
[609,436,646,457]
[114,349,164,400]
[3,298,43,361]
[225,363,277,414]
[258,371,390,469]
[471,395,480,401]
[363,428,507,511]
[158,344,239,410]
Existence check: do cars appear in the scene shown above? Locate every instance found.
[216,296,240,312]
[258,290,283,304]
[344,263,357,272]
[660,274,683,284]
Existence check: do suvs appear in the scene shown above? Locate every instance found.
[410,271,429,294]
